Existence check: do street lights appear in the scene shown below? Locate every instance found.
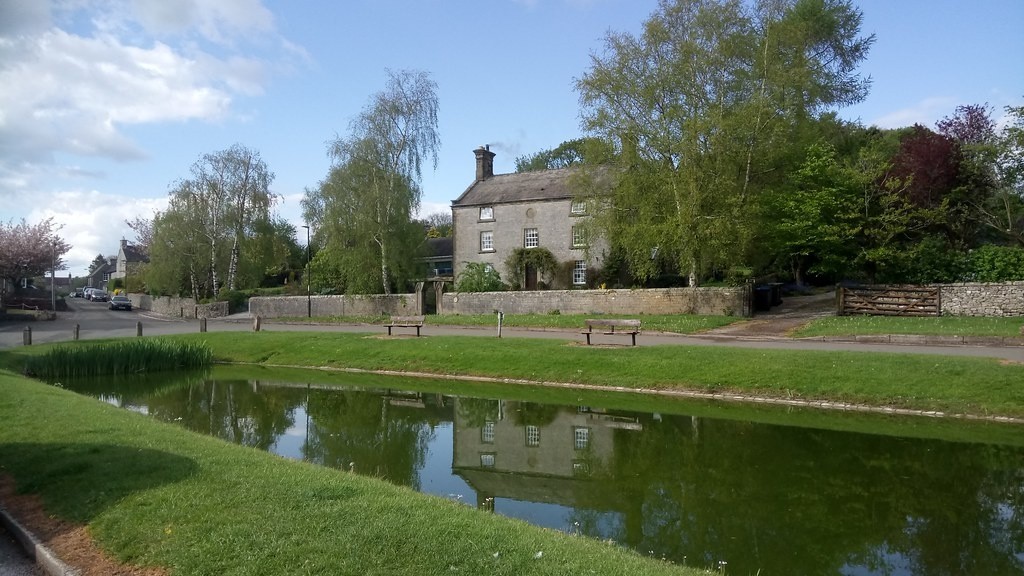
[301,225,314,319]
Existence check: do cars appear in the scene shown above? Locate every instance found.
[74,286,109,303]
[108,296,132,311]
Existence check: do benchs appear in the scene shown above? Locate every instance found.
[382,315,426,337]
[581,319,641,347]
